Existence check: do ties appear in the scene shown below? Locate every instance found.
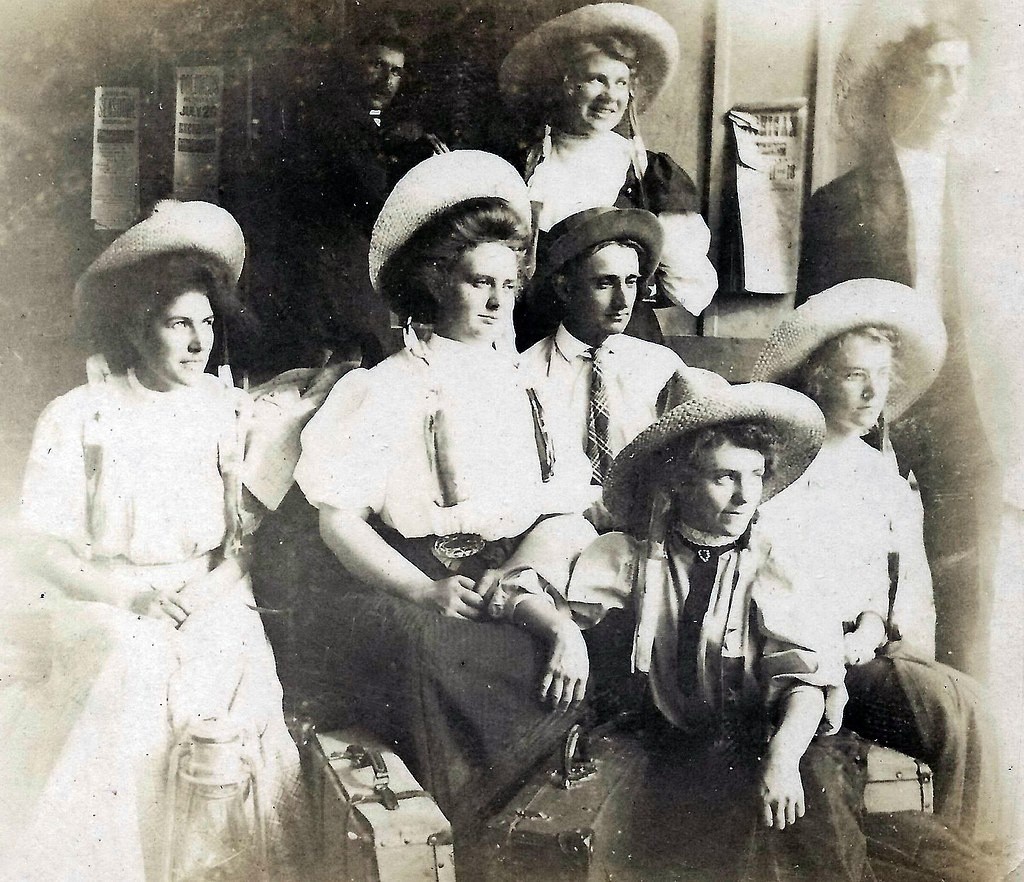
[586,346,613,485]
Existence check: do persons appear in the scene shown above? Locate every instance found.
[296,1,1000,818]
[751,275,1013,881]
[0,197,362,882]
[486,367,872,881]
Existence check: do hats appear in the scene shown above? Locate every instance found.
[538,207,665,294]
[72,201,245,343]
[834,0,999,147]
[602,383,827,533]
[498,3,679,117]
[750,278,948,435]
[368,150,532,293]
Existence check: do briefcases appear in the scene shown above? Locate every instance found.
[485,720,650,882]
[311,725,456,882]
[830,726,934,814]
